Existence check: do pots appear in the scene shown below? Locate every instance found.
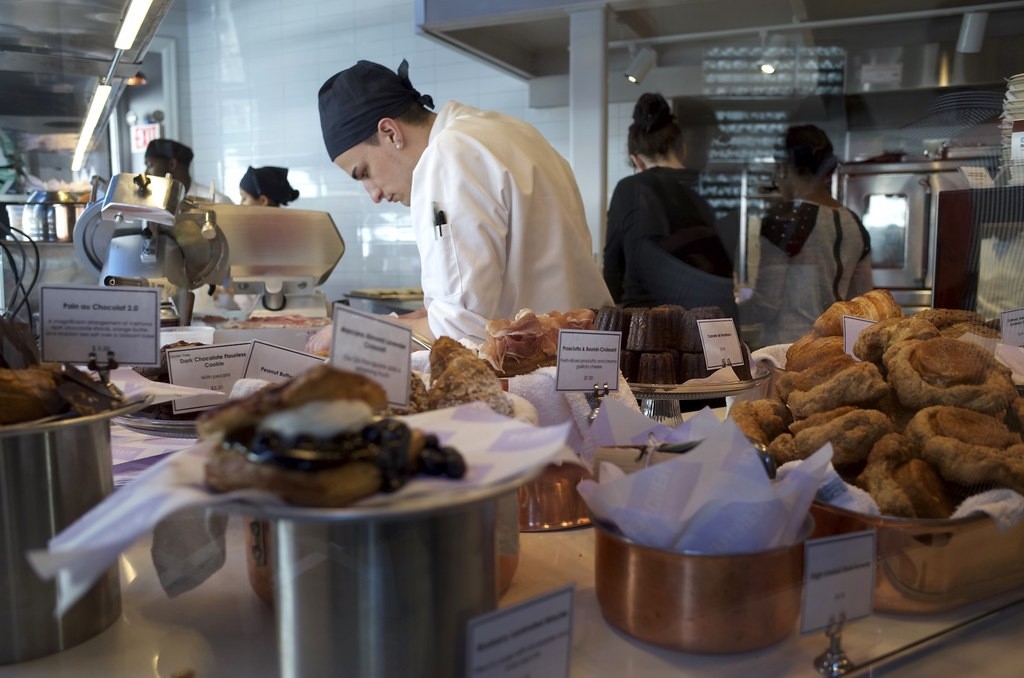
[0,394,161,666]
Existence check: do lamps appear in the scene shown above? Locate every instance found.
[755,28,792,77]
[71,0,152,170]
[954,8,990,58]
[622,43,657,88]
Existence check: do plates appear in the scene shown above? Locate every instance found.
[629,360,774,429]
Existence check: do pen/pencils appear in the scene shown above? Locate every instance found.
[437,210,446,237]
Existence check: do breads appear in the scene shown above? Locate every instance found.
[731,286,1024,523]
[387,336,517,418]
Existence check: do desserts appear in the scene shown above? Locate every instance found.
[592,303,751,386]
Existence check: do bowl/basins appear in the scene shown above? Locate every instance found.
[202,378,1024,677]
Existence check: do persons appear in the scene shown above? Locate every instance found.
[735,124,873,346]
[603,92,741,337]
[146,140,234,310]
[212,167,299,310]
[306,59,615,360]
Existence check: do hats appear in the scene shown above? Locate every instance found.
[144,139,194,168]
[239,166,300,207]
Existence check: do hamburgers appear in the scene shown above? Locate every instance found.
[195,364,392,509]
